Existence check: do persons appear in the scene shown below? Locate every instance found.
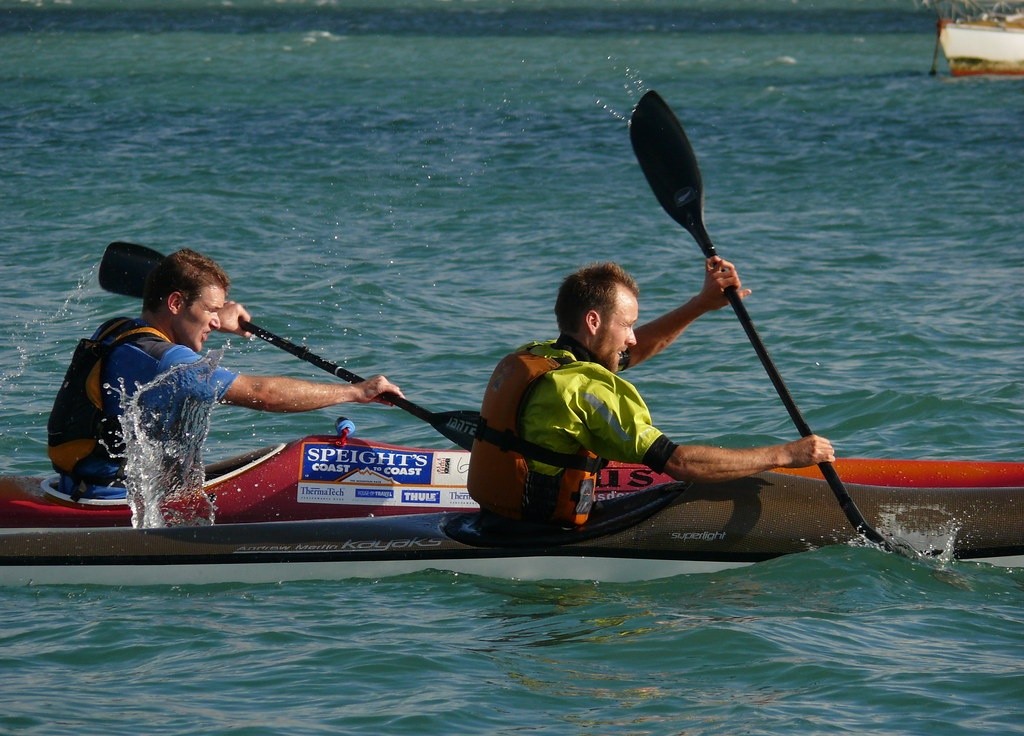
[468,256,836,544]
[47,248,407,501]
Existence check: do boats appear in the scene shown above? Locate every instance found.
[0,416,1022,566]
[938,15,1024,77]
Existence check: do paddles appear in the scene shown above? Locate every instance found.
[625,85,902,555]
[102,240,482,452]
